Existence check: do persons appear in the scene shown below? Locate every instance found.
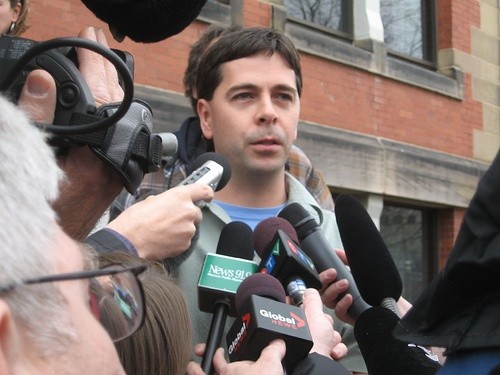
[18,26,125,241]
[163,28,369,375]
[0,95,500,375]
[0,0,32,37]
[109,21,335,225]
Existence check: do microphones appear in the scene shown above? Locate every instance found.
[177,160,224,210]
[354,306,442,375]
[277,202,372,319]
[333,193,403,322]
[225,273,314,375]
[198,221,254,375]
[253,216,323,309]
[192,152,232,192]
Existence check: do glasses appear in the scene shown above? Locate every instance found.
[0,262,148,342]
